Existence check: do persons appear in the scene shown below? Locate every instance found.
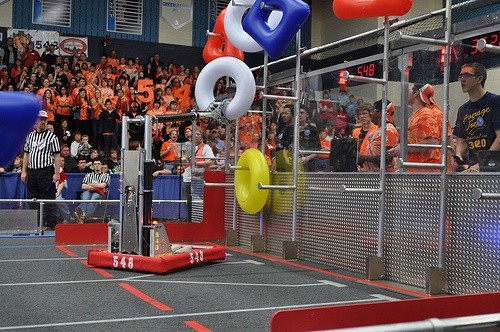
[73,158,111,224]
[281,104,321,172]
[386,81,455,173]
[358,99,399,172]
[138,53,294,176]
[182,129,216,223]
[352,103,378,172]
[21,111,61,231]
[309,89,364,173]
[0,34,144,174]
[450,62,500,173]
[53,163,70,224]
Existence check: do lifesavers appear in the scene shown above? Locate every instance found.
[234,147,271,215]
[202,8,244,63]
[194,56,257,122]
[266,150,305,214]
[242,0,310,60]
[224,0,283,53]
[333,0,413,20]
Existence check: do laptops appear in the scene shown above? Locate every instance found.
[478,150,500,173]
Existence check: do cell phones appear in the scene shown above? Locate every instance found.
[452,155,469,165]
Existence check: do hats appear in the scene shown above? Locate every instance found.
[413,80,437,105]
[375,99,395,122]
[38,110,48,118]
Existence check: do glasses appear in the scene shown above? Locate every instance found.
[458,73,479,79]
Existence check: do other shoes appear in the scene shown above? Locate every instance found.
[74,209,85,224]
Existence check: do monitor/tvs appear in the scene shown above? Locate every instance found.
[330,138,359,172]
